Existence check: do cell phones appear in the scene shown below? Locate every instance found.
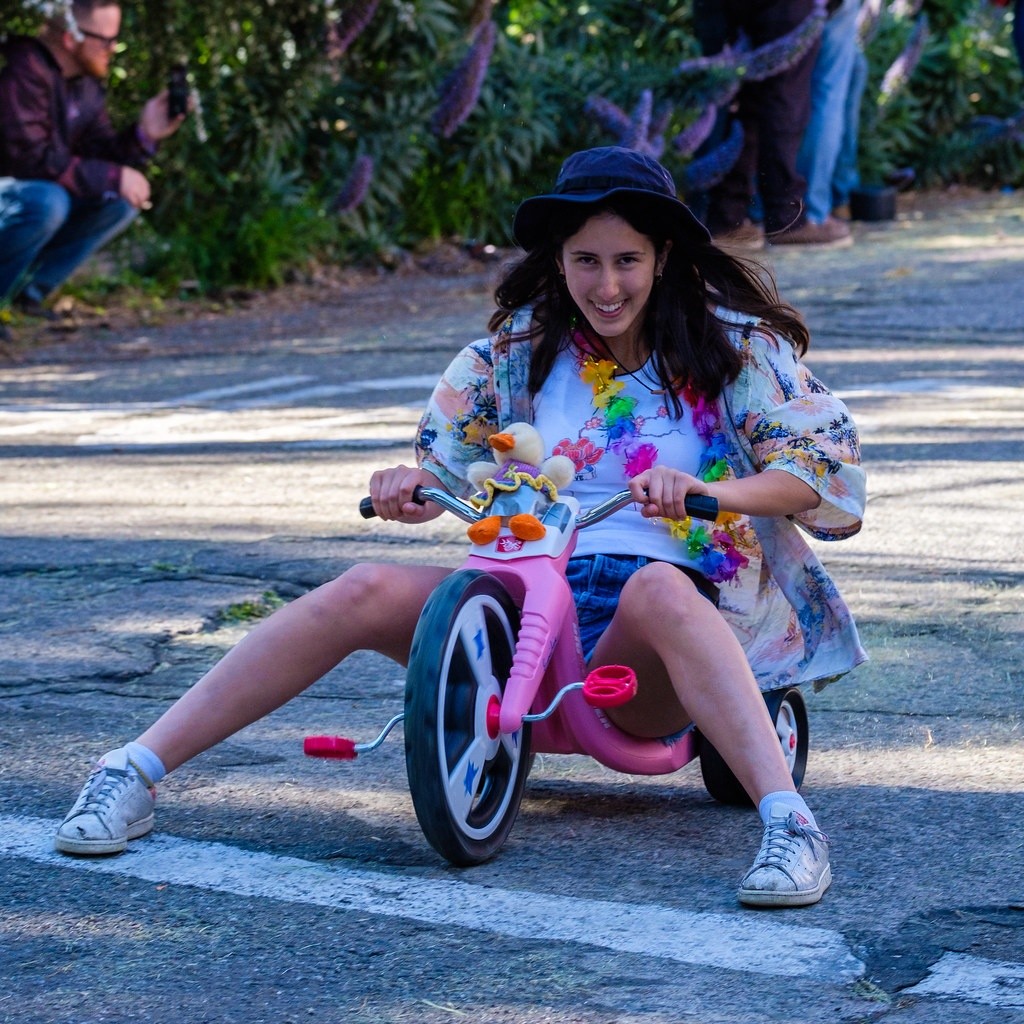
[168,63,187,119]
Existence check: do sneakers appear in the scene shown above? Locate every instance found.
[736,800,833,906]
[55,747,155,855]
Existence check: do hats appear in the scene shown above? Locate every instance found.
[511,145,711,258]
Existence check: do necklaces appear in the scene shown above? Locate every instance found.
[572,323,749,588]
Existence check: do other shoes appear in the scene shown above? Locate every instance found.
[15,291,111,330]
[0,306,53,344]
[763,206,855,251]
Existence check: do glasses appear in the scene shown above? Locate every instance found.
[75,26,124,52]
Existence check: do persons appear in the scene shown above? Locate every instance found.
[0,0,201,342]
[54,145,868,906]
[623,0,882,254]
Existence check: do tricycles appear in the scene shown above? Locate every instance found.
[300,484,812,869]
